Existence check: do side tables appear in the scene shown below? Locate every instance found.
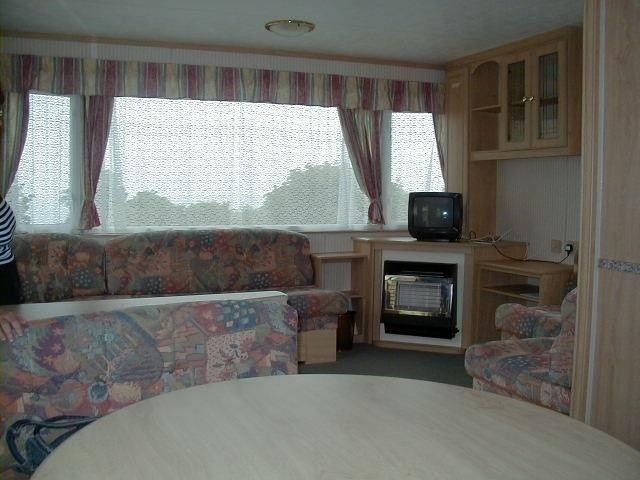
[310,251,366,344]
[472,257,577,346]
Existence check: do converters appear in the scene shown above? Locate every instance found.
[565,244,572,254]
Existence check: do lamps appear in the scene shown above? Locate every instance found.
[265,21,314,38]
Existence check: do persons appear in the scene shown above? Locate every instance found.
[0,196,21,305]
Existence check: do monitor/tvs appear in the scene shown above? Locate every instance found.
[407,192,464,242]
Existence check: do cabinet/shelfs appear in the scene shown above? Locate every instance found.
[469,24,584,164]
[444,55,496,241]
[351,234,530,356]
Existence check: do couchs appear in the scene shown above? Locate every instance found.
[495,289,577,345]
[0,290,299,476]
[464,288,576,417]
[109,228,346,364]
[13,232,105,304]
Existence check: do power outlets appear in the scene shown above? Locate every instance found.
[563,240,579,257]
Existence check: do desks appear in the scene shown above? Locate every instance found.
[28,374,640,480]
